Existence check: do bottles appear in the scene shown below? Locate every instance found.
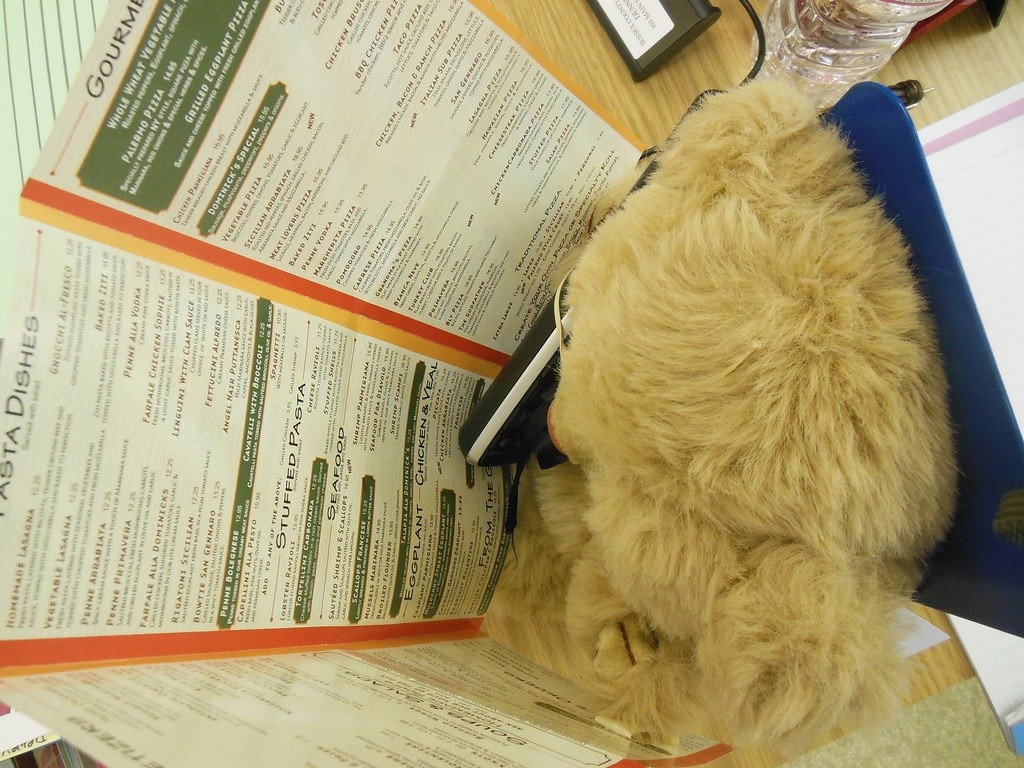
[750,0,952,110]
